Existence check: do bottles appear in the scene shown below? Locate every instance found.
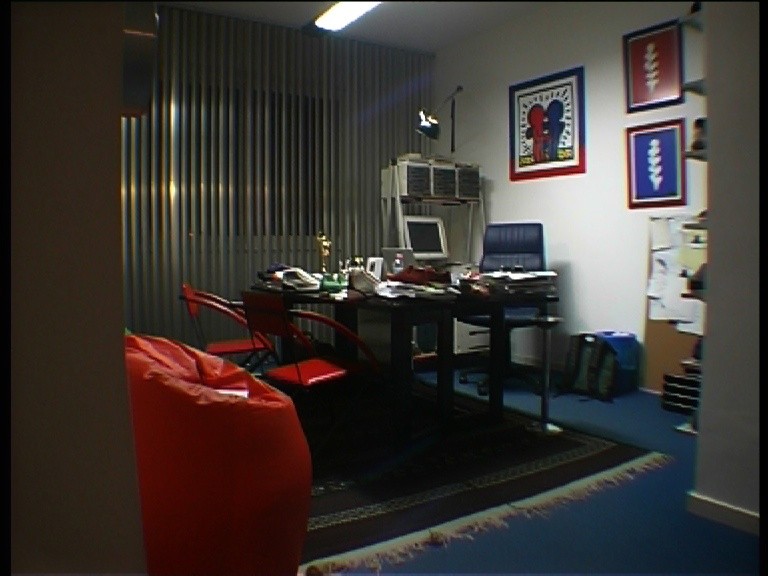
[393,253,403,275]
[337,255,364,284]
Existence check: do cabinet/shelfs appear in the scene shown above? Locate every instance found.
[678,2,709,303]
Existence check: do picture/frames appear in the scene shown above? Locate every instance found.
[622,18,685,114]
[508,63,587,182]
[625,117,688,210]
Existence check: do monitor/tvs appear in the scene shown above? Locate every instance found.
[402,215,450,259]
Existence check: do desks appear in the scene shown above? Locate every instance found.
[250,282,566,447]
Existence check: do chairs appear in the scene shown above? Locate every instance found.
[125,333,312,576]
[459,222,549,396]
[237,290,380,455]
[178,280,276,370]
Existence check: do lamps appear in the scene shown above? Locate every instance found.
[415,84,463,152]
[312,2,385,32]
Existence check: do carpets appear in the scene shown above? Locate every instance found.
[303,383,670,576]
